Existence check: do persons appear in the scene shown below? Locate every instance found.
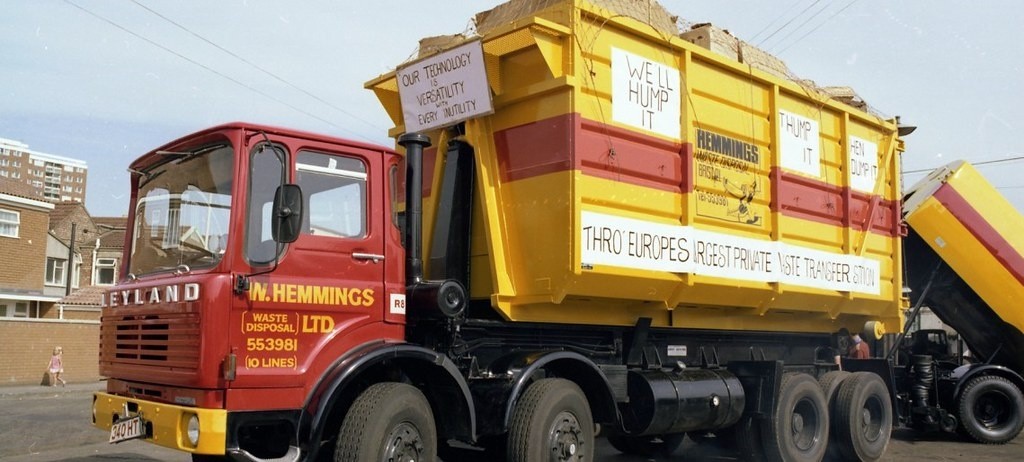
[847,333,869,359]
[46,346,66,388]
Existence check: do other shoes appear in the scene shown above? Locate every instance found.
[51,384,56,387]
[63,381,66,387]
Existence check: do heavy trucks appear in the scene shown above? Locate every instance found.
[93,0,910,462]
[893,161,1024,443]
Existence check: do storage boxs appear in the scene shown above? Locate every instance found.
[419,1,855,103]
